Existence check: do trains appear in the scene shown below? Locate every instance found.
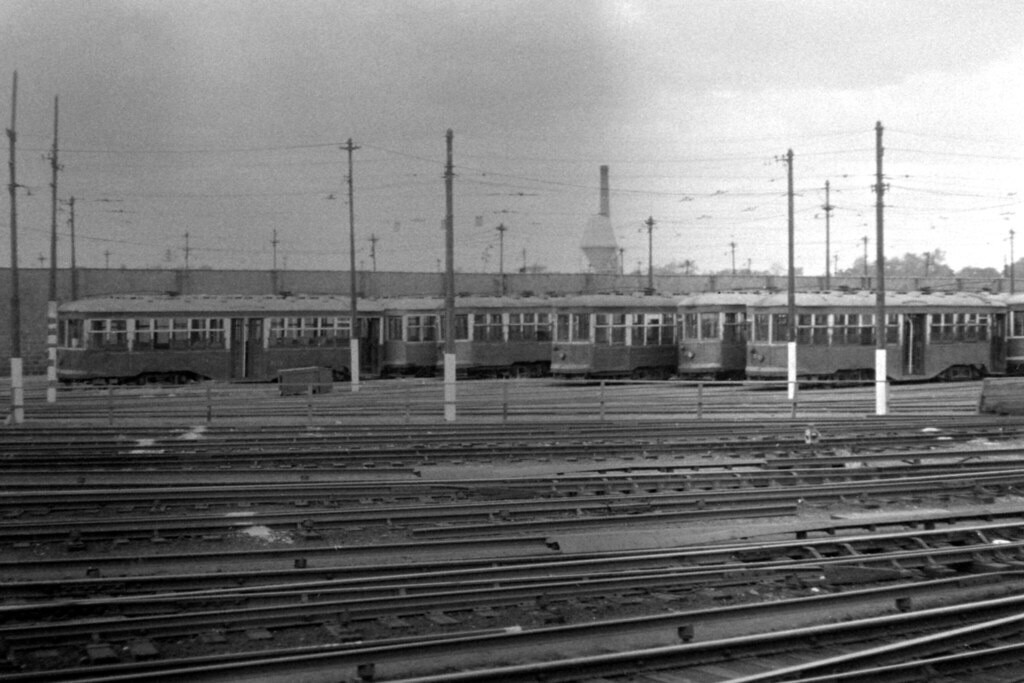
[57,292,1024,389]
